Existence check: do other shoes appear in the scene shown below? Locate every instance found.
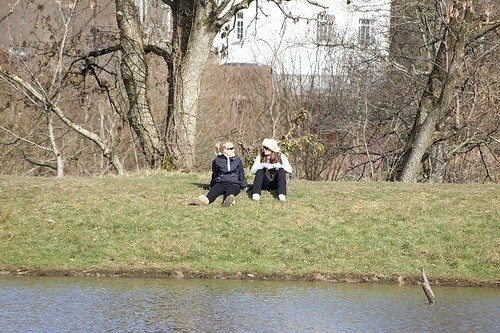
[252,194,260,201]
[278,194,286,202]
[191,196,209,206]
[222,195,235,207]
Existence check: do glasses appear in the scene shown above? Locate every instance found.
[226,147,235,151]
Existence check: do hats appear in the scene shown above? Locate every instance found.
[262,138,280,153]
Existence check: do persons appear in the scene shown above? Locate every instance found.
[251,139,292,202]
[192,142,249,206]
